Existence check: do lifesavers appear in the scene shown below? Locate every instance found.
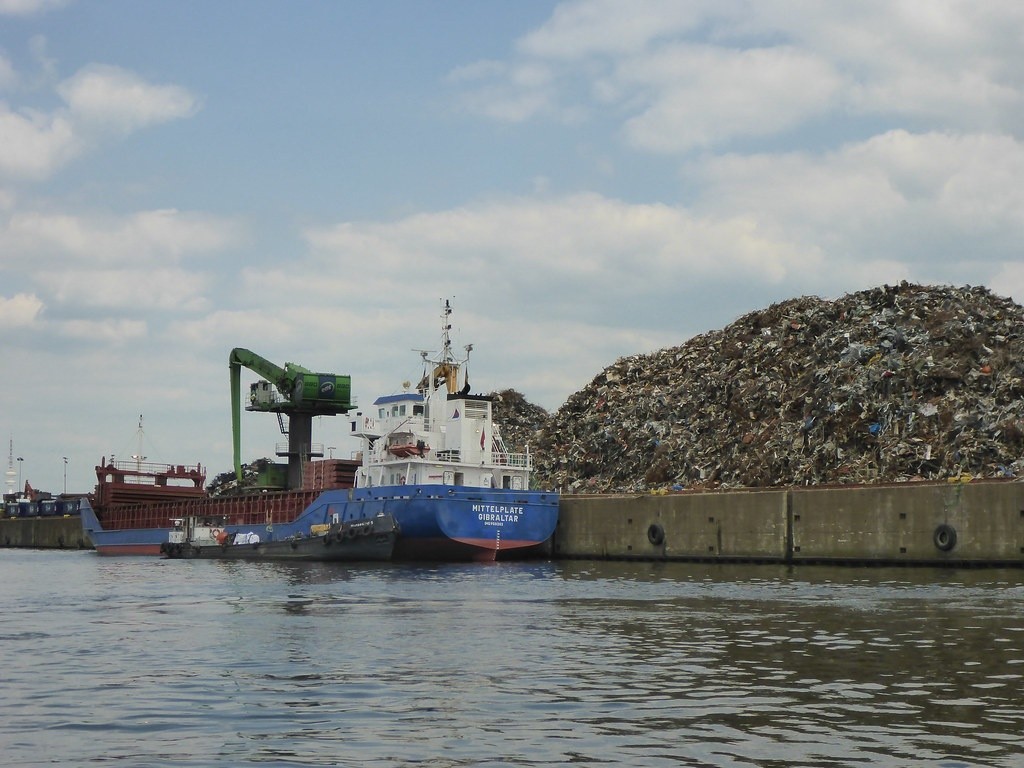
[212,528,221,538]
[647,523,665,546]
[400,476,406,483]
[335,532,344,544]
[290,542,297,549]
[347,528,358,540]
[365,418,372,429]
[322,535,330,547]
[934,524,957,552]
[362,525,373,536]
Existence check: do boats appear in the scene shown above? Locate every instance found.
[389,439,431,458]
[78,292,561,564]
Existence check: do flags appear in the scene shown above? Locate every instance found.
[480,427,485,449]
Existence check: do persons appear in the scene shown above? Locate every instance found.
[266,522,273,541]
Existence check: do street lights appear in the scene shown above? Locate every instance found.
[16,456,24,504]
[62,456,69,493]
